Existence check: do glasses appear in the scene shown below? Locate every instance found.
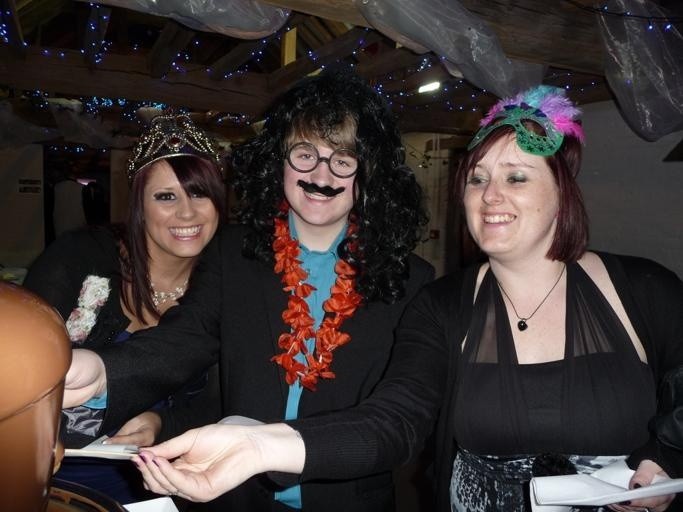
[285,140,359,178]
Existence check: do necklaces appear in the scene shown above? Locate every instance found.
[142,275,187,318]
[486,266,572,330]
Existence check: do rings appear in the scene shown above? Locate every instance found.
[166,489,181,497]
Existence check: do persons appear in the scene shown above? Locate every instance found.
[58,70,444,510]
[16,102,227,510]
[126,82,683,512]
